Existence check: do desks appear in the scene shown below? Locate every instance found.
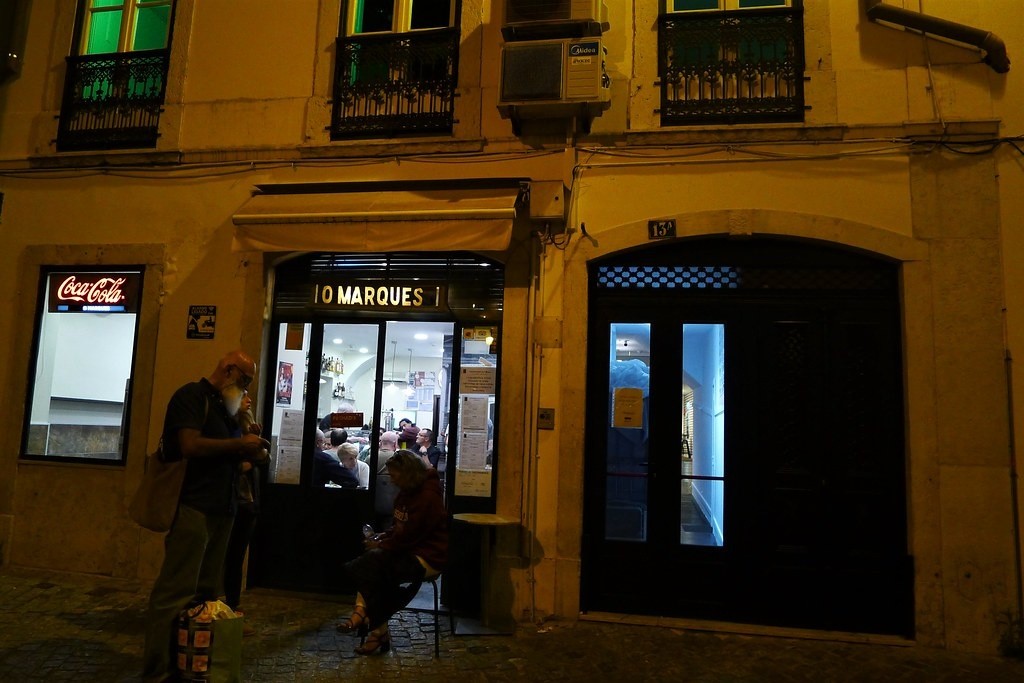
[452,512,521,635]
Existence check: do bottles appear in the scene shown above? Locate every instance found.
[334,382,345,399]
[361,522,378,541]
[369,417,373,430]
[322,353,344,374]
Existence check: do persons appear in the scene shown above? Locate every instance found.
[315,402,494,490]
[336,449,457,658]
[141,349,270,683]
[197,387,272,637]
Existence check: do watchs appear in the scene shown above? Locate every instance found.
[377,539,383,548]
[421,452,428,456]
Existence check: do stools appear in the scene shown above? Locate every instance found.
[360,567,442,658]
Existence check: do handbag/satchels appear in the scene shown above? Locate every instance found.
[176,600,245,683]
[128,450,187,533]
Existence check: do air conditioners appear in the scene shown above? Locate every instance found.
[497,41,612,117]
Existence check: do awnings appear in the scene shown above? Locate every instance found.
[230,186,521,253]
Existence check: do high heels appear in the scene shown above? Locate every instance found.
[335,611,369,636]
[354,631,391,652]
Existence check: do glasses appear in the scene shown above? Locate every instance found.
[232,364,249,384]
[417,433,428,437]
[394,451,402,464]
